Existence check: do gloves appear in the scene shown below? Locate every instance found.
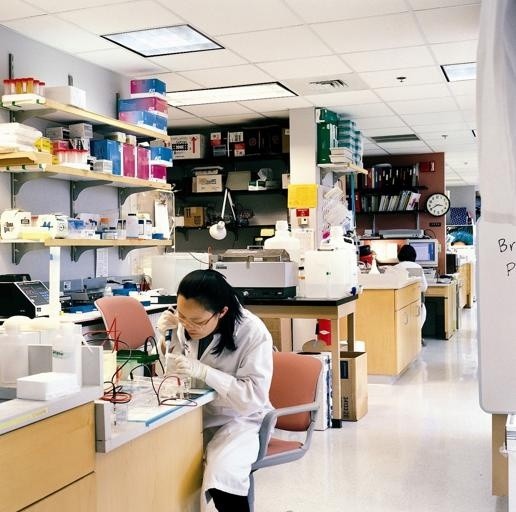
[157,310,180,348]
[174,355,210,383]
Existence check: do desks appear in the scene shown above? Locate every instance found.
[238,293,359,429]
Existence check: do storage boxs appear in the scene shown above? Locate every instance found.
[44,86,87,109]
[450,207,466,225]
[183,206,207,228]
[90,78,223,193]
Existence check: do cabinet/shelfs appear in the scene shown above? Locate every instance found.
[352,185,427,214]
[166,152,290,229]
[0,387,218,512]
[357,261,475,376]
[0,94,172,263]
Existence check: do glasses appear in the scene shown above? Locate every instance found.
[176,311,215,331]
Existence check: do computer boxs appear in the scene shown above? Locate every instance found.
[423,270,436,279]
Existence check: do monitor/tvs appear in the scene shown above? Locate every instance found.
[406,239,439,268]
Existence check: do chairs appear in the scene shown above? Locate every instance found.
[247,351,324,512]
[94,296,157,350]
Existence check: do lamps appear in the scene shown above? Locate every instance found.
[209,187,237,240]
[286,183,318,225]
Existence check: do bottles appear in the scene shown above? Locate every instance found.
[98,212,153,240]
[2,77,46,98]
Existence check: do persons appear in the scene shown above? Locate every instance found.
[149,270,273,512]
[394,244,428,347]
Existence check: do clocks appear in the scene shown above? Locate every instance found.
[425,192,450,217]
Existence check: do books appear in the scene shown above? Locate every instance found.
[346,163,421,210]
